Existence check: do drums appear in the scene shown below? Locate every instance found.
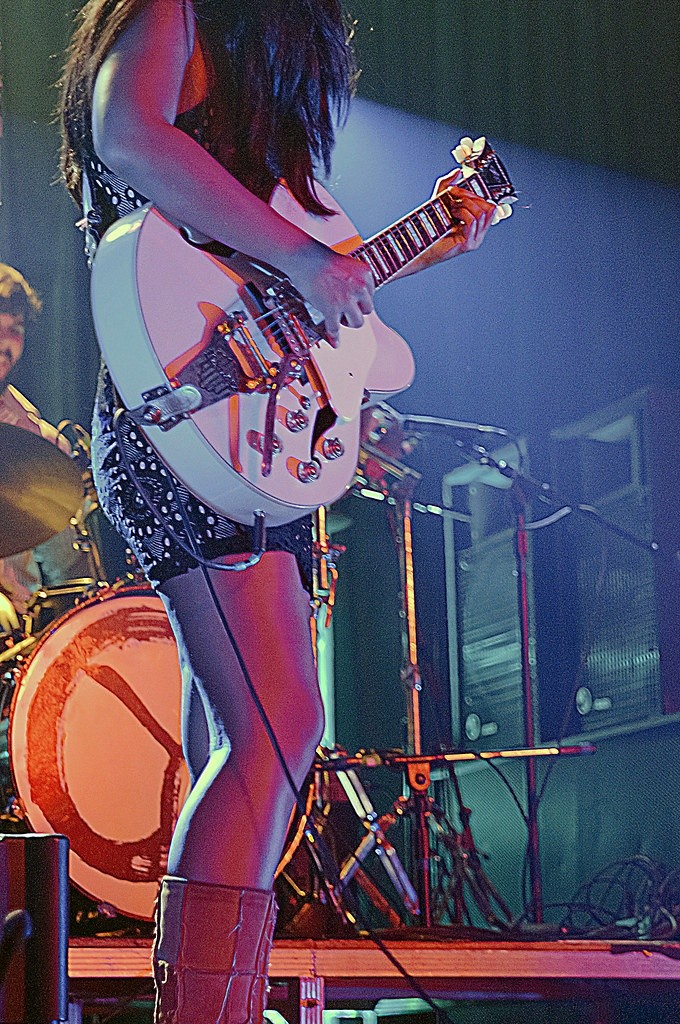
[6,582,196,926]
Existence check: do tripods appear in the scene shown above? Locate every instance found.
[283,453,510,941]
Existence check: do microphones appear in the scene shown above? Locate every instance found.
[402,415,508,444]
[73,424,92,461]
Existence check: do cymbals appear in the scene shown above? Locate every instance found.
[0,420,89,560]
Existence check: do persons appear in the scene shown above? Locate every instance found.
[0,265,100,648]
[56,0,517,1024]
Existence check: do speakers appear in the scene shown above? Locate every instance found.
[402,387,680,940]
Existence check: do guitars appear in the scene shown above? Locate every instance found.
[85,132,521,533]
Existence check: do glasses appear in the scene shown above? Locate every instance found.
[0,325,25,342]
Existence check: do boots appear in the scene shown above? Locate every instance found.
[149,876,280,1024]
